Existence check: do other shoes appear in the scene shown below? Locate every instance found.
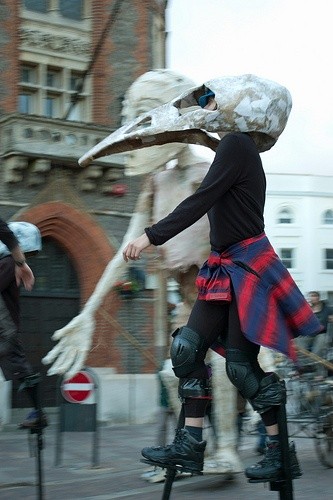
[20,412,47,427]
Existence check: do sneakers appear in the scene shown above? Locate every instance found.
[244,439,302,480]
[142,428,206,472]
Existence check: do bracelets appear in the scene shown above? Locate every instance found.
[15,259,24,267]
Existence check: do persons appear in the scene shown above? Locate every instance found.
[153,389,246,457]
[43,70,277,481]
[0,210,34,290]
[123,97,323,479]
[272,291,333,357]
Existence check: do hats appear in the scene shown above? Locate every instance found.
[198,91,214,107]
